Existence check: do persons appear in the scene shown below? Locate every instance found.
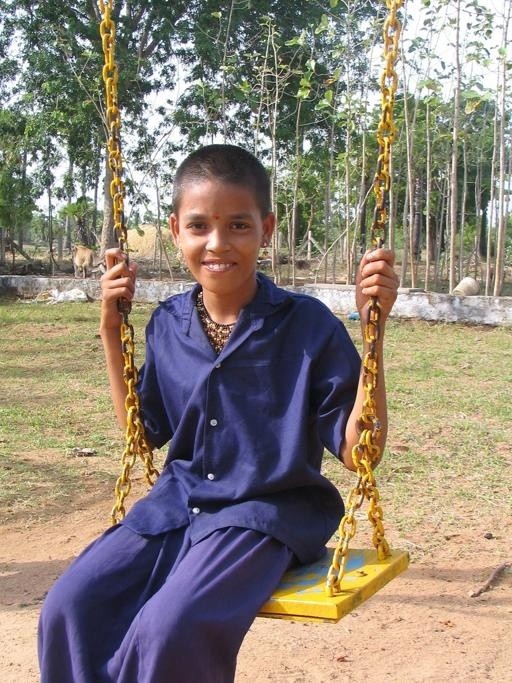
[36,145,400,683]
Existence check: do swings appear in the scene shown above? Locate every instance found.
[93,0,410,624]
